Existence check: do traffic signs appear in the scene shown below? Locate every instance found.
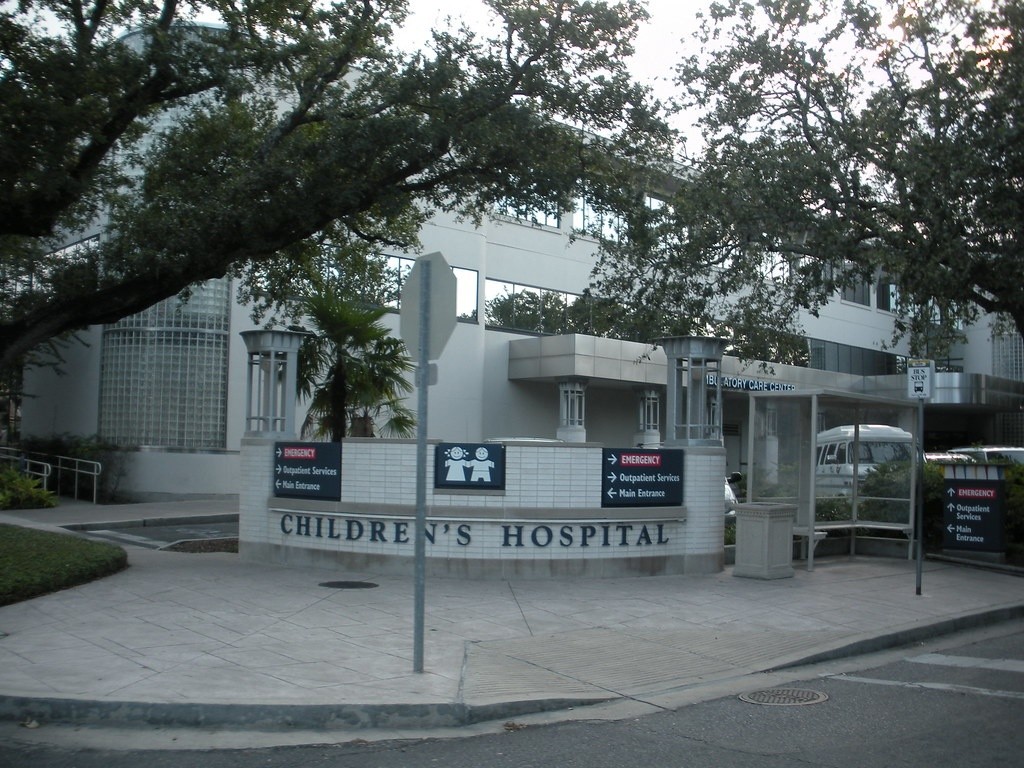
[943,479,1005,550]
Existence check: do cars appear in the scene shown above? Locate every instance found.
[724,472,743,517]
[923,451,977,463]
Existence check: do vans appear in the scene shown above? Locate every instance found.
[950,447,1023,462]
[815,424,927,500]
